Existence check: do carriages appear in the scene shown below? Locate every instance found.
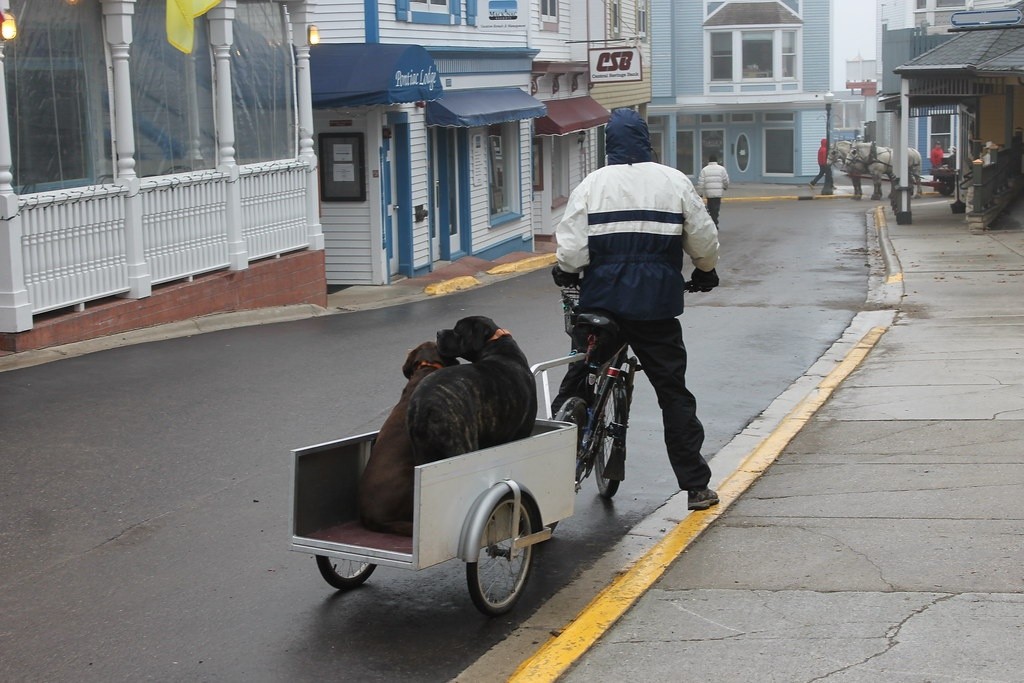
[826,139,956,201]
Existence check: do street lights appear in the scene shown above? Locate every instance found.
[824,91,834,196]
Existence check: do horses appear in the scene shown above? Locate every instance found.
[826,140,923,200]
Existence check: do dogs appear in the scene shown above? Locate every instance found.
[358,340,461,539]
[406,316,537,467]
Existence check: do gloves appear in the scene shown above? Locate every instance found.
[552,265,580,288]
[723,188,727,190]
[691,268,719,293]
[700,194,703,197]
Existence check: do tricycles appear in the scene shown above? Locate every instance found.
[286,268,702,614]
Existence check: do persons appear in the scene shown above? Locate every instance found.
[808,139,837,190]
[550,106,722,511]
[696,153,729,230]
[930,139,944,192]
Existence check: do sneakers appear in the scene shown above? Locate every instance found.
[687,488,720,509]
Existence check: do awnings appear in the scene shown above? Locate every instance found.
[533,96,612,136]
[426,85,549,131]
[294,43,442,107]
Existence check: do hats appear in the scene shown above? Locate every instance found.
[935,140,941,144]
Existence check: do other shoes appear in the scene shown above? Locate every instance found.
[809,182,815,190]
[833,187,836,190]
